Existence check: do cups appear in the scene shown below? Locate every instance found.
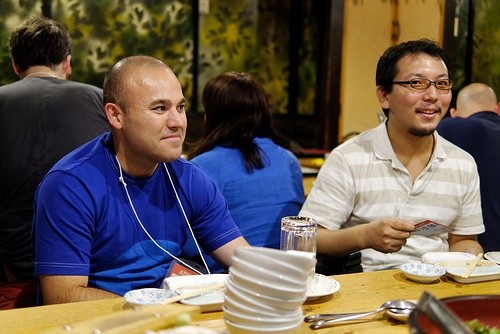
[280,216,317,294]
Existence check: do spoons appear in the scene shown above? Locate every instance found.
[304,300,416,330]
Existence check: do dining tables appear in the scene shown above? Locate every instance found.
[0,263,500,334]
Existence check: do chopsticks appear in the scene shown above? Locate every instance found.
[462,253,483,278]
[160,283,225,306]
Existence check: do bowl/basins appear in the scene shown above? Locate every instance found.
[407,294,500,334]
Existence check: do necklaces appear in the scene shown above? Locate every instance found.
[115,157,211,275]
[21,71,59,81]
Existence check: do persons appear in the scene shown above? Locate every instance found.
[31,54,255,308]
[1,16,113,285]
[297,37,486,272]
[436,82,500,255]
[185,71,306,275]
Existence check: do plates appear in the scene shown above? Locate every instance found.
[157,325,218,334]
[221,246,317,334]
[124,288,178,309]
[400,263,446,283]
[306,273,340,300]
[483,251,500,264]
[163,274,229,312]
[422,252,500,283]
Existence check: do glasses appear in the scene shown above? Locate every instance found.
[393,78,454,90]
